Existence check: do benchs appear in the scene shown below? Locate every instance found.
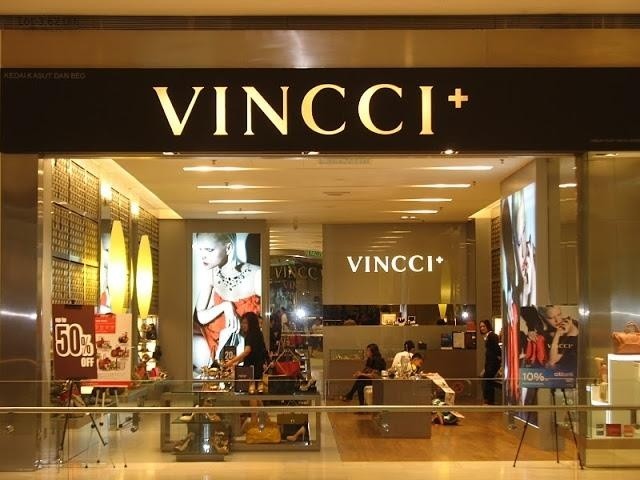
[364,385,373,405]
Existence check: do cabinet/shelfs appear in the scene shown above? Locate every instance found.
[51,327,322,460]
[371,380,433,438]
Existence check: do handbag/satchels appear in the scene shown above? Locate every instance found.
[146,323,158,340]
[611,321,640,354]
[246,423,281,444]
[274,349,300,378]
[234,365,253,390]
[277,400,308,425]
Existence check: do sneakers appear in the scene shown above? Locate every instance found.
[258,380,265,394]
[288,332,303,345]
[248,380,256,394]
[339,395,352,402]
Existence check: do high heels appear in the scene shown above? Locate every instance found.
[208,438,229,453]
[174,438,192,451]
[287,426,305,442]
[299,377,316,392]
[180,404,201,421]
[202,402,221,422]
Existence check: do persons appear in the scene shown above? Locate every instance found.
[193,232,262,361]
[518,304,579,372]
[311,318,323,329]
[339,343,386,415]
[391,340,416,370]
[502,187,536,404]
[479,320,501,405]
[270,303,289,352]
[223,312,271,425]
[411,353,425,368]
